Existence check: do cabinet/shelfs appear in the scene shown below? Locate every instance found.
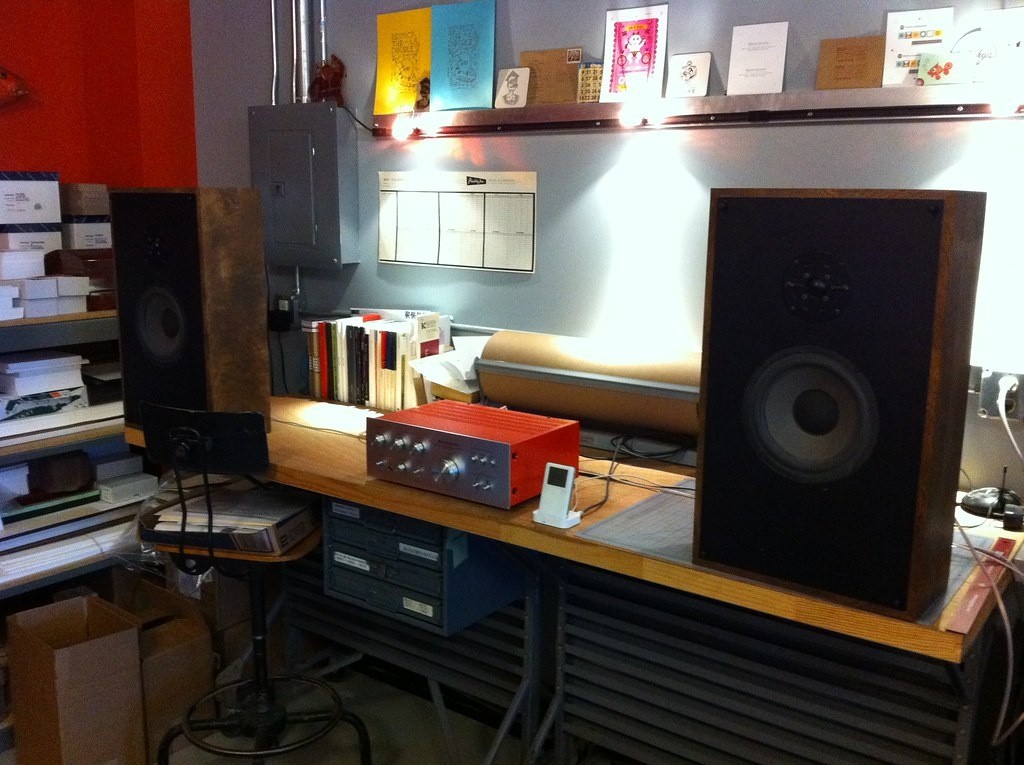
[0,309,247,620]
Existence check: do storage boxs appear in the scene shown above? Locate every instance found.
[143,565,288,716]
[95,474,159,504]
[77,567,216,765]
[0,183,116,321]
[5,594,147,765]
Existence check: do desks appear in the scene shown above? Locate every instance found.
[124,395,1024,765]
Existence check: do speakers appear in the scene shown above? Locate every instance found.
[110,187,271,435]
[693,187,988,623]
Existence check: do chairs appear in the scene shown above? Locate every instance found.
[137,399,373,765]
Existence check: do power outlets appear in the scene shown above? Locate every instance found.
[978,369,1024,422]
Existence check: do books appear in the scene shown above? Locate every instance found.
[308,313,451,412]
